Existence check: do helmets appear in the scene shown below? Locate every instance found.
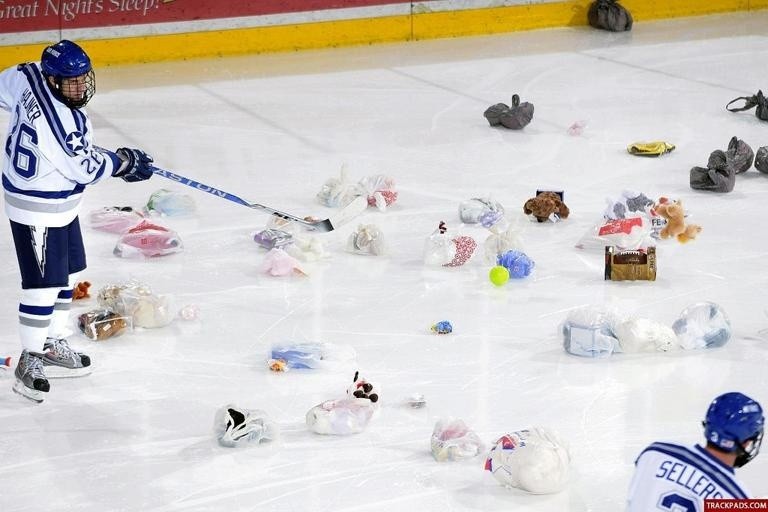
[704,392,765,468]
[40,40,96,108]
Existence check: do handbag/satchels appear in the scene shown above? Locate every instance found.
[588,2,633,31]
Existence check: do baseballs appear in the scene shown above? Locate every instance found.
[489,265,511,288]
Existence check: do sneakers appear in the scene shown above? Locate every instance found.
[42,337,91,368]
[15,348,50,392]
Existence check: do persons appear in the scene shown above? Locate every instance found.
[623,392,764,512]
[0,39,153,391]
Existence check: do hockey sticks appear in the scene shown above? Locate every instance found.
[91,146,368,234]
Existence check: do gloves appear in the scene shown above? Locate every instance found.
[113,147,154,182]
